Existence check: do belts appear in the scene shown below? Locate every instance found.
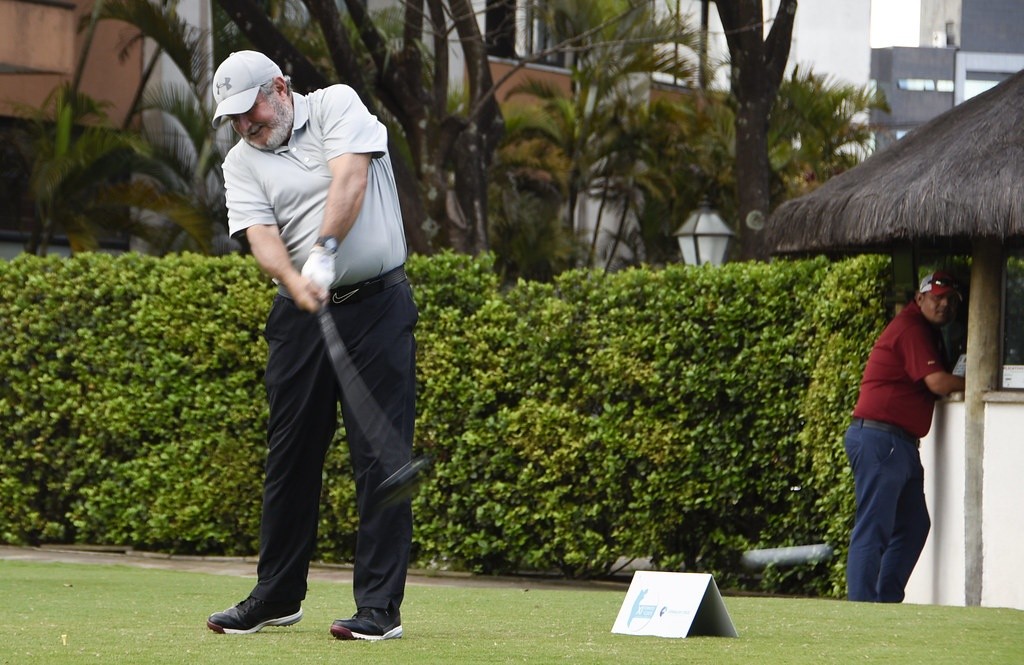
[851,417,920,447]
[278,262,407,304]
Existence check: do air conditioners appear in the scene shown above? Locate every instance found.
[932,31,948,49]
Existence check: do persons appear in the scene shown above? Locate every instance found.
[204,49,419,643]
[845,270,966,603]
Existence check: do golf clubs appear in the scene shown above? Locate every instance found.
[317,306,430,496]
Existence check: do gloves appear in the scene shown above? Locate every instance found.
[299,245,338,305]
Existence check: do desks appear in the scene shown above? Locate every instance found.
[901,386,1024,610]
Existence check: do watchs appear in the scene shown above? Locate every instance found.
[314,236,341,252]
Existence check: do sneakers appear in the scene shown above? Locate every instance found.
[207,594,304,634]
[330,607,403,639]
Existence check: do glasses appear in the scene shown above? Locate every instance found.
[922,277,962,288]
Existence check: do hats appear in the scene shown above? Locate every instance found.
[211,50,283,128]
[919,270,965,301]
